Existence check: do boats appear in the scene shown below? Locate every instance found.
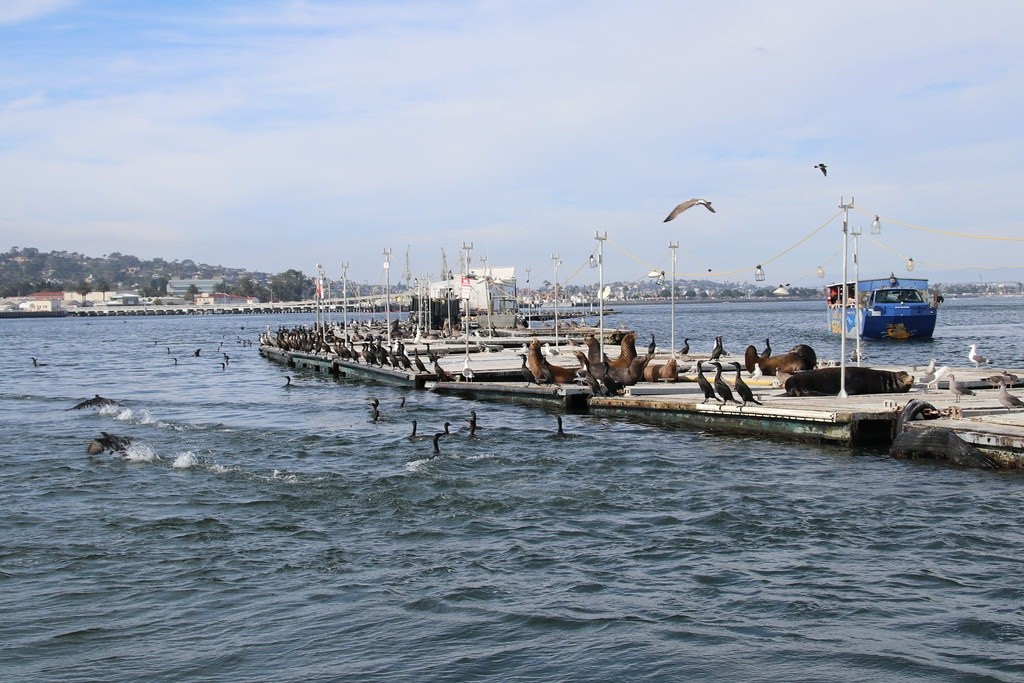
[826,272,939,343]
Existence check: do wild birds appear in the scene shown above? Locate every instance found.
[676,336,772,359]
[27,317,541,459]
[584,359,621,398]
[697,360,763,406]
[815,164,828,176]
[552,413,566,438]
[541,355,555,387]
[915,344,1024,413]
[664,198,717,222]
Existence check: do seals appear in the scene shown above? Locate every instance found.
[529,337,574,383]
[745,344,816,375]
[785,367,914,396]
[573,333,679,383]
